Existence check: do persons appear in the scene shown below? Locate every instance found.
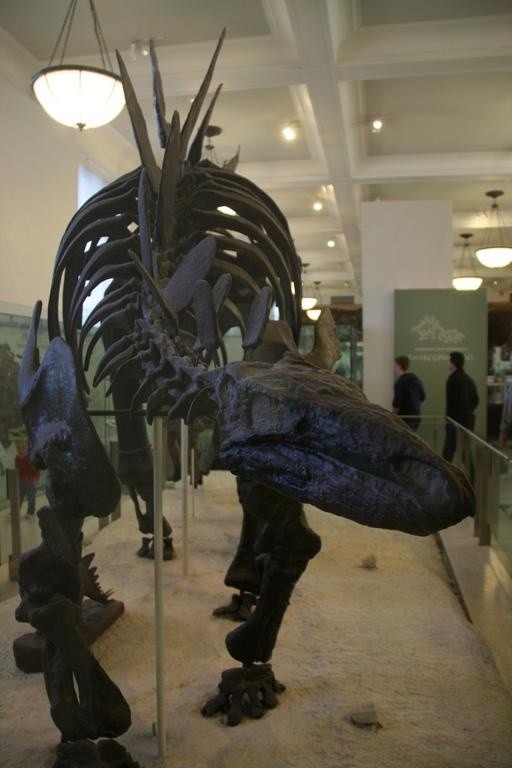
[389,357,427,434]
[498,382,511,448]
[438,353,479,485]
[166,430,182,482]
[8,437,42,520]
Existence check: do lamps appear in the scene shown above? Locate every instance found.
[301,262,323,322]
[449,189,512,292]
[30,0,127,132]
